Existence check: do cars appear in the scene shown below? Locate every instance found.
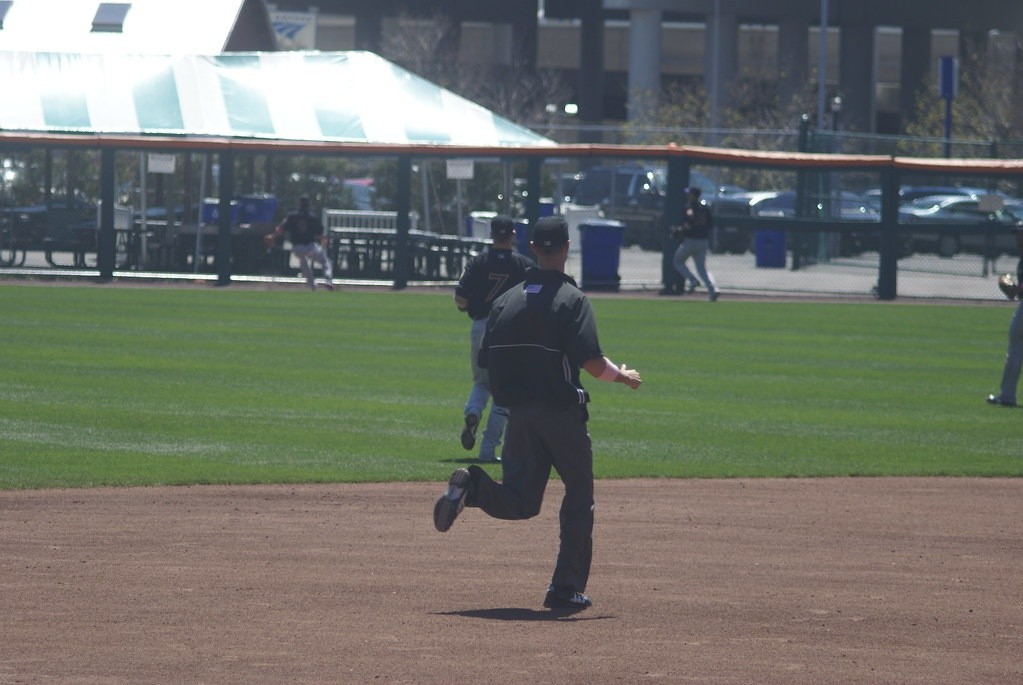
[542,165,1022,260]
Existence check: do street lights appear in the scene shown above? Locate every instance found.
[829,94,842,134]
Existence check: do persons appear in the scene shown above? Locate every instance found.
[986,220,1023,407]
[435,214,642,609]
[264,194,337,290]
[660,188,720,301]
[460,216,537,462]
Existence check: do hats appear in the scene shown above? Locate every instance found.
[534,216,569,245]
[685,187,700,196]
[492,216,513,236]
[300,195,309,205]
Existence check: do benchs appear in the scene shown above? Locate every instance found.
[0,238,84,270]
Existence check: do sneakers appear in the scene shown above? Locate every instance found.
[461,414,476,449]
[482,457,501,464]
[324,281,334,290]
[710,289,719,301]
[544,584,592,608]
[308,284,318,289]
[434,469,471,532]
[686,281,701,293]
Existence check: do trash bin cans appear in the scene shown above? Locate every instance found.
[576,220,628,290]
[237,193,278,224]
[754,210,786,268]
[203,197,239,223]
[524,196,556,216]
[512,218,529,257]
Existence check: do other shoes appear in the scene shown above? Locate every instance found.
[987,394,1015,406]
